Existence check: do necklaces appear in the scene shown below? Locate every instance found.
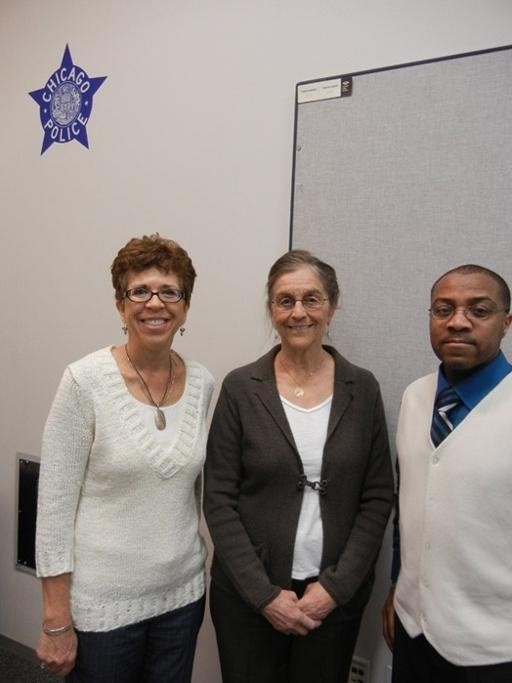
[276,349,328,399]
[125,343,175,432]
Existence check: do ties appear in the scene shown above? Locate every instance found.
[431,389,461,449]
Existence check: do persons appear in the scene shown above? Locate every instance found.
[379,261,512,681]
[203,248,393,683]
[33,231,219,683]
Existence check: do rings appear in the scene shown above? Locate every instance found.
[39,664,46,669]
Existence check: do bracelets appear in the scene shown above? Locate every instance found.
[40,618,74,636]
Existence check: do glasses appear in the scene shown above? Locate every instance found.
[274,293,329,309]
[122,287,185,303]
[428,304,504,321]
